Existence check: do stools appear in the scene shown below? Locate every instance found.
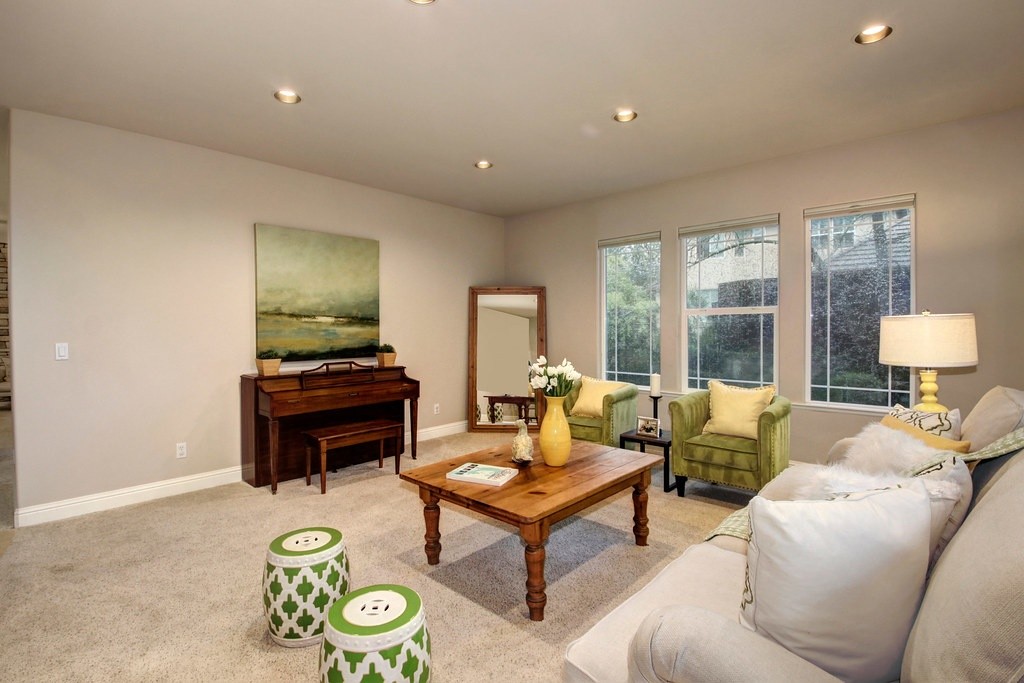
[477,404,482,423]
[262,527,432,683]
[487,404,503,422]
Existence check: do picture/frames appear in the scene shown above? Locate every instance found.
[636,416,659,438]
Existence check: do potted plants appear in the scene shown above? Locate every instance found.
[376,344,397,367]
[256,349,281,376]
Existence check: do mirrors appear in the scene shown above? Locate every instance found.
[468,286,547,434]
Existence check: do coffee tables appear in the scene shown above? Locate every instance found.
[400,434,665,622]
[483,396,538,425]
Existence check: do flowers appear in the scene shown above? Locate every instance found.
[527,354,582,397]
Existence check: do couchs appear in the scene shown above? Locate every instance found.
[564,382,638,450]
[668,390,791,498]
[563,383,1024,683]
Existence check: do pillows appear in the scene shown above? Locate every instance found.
[702,379,776,440]
[570,375,627,419]
[739,402,973,683]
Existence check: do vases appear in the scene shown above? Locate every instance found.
[539,395,571,466]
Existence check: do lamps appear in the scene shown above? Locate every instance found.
[879,308,978,412]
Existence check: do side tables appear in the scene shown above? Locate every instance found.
[619,429,688,492]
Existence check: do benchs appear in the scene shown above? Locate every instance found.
[301,415,404,494]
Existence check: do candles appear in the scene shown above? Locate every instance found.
[650,372,660,396]
